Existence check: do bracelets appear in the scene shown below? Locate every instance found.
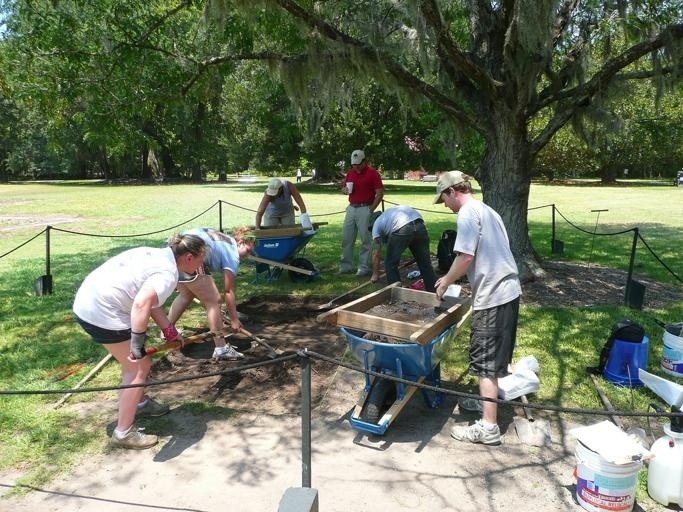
[160,322,179,341]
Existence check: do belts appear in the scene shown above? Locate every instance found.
[407,219,423,225]
[350,203,369,208]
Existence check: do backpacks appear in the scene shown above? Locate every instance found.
[436,230,456,271]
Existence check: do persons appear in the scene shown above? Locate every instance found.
[296,168,302,182]
[329,149,384,277]
[70,232,208,451]
[253,177,319,237]
[366,205,439,294]
[160,225,256,363]
[432,168,524,446]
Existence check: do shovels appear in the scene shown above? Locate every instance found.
[129,287,285,360]
[314,258,417,310]
[506,362,552,447]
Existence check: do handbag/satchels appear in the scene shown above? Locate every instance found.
[598,319,644,372]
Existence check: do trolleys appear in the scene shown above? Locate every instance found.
[244,227,320,286]
[335,282,474,439]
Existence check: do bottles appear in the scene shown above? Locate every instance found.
[411,279,425,289]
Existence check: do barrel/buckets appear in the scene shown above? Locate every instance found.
[603,335,649,388]
[660,330,683,379]
[647,436,683,509]
[575,440,643,512]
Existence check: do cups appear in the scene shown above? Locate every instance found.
[346,181,354,194]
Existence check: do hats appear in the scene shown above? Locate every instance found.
[367,211,383,227]
[433,170,465,204]
[267,179,283,197]
[350,149,365,166]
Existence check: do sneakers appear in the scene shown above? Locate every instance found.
[212,342,245,362]
[110,424,159,450]
[136,395,169,416]
[161,325,193,338]
[458,396,483,412]
[176,333,177,335]
[452,420,501,445]
[356,269,366,276]
